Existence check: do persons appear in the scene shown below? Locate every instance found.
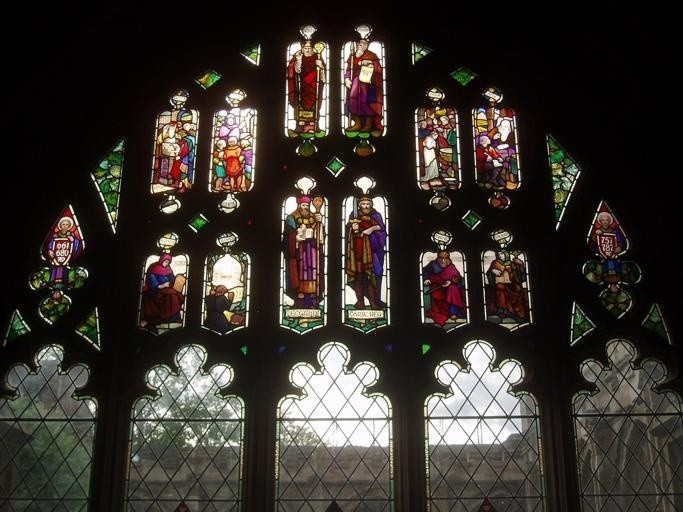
[343,197,387,310]
[342,40,382,132]
[485,250,528,321]
[421,252,467,324]
[142,255,184,325]
[155,112,252,194]
[415,108,521,189]
[203,285,234,336]
[286,40,326,137]
[280,196,324,309]
[47,217,78,300]
[591,212,625,284]
